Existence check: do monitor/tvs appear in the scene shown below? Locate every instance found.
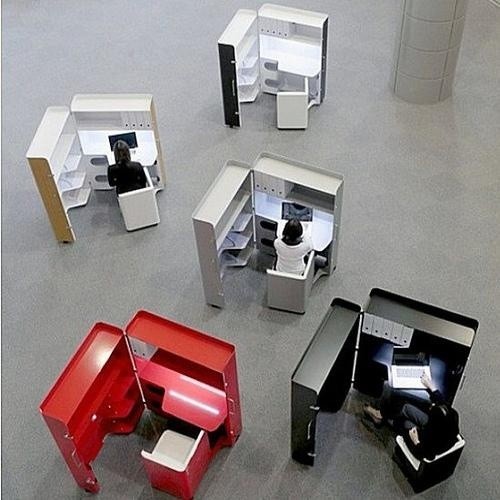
[108,131,138,151]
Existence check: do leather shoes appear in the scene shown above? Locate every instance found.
[363,402,382,425]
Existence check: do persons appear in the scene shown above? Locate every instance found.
[106,139,147,195]
[272,220,312,276]
[362,367,462,460]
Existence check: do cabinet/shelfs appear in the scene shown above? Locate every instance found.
[186,147,346,315]
[211,2,333,131]
[21,87,171,246]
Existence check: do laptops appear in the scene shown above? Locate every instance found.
[391,346,432,390]
[278,201,313,235]
